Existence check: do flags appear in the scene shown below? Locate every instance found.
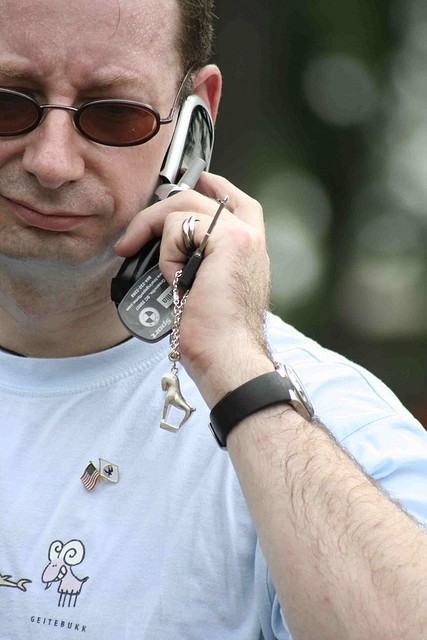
[80,462,99,491]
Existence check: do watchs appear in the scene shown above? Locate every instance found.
[205,360,316,450]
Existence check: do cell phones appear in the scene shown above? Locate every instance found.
[109,95,215,306]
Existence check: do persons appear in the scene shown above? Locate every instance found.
[0,1,426,639]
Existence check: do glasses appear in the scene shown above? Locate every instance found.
[0,61,196,148]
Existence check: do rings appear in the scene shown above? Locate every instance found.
[181,214,200,253]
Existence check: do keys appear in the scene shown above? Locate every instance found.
[175,194,230,291]
